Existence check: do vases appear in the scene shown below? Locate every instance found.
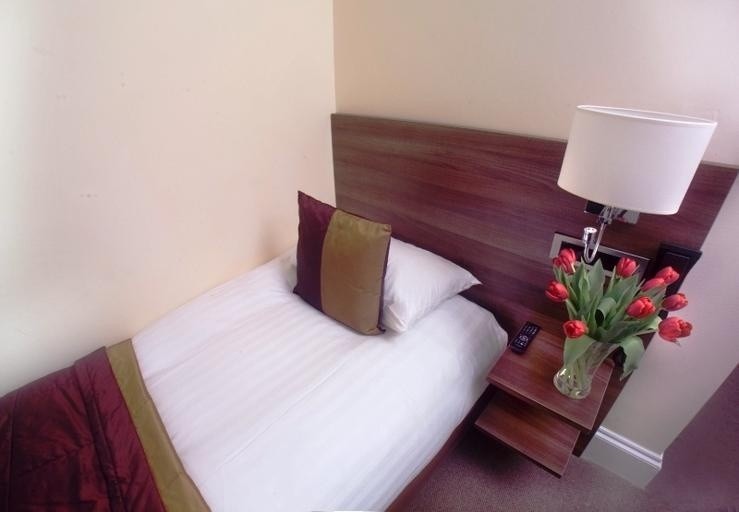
[553,332,623,401]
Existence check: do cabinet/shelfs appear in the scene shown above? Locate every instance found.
[475,328,615,477]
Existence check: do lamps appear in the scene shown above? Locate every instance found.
[556,103,718,265]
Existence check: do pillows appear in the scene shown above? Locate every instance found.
[381,238,483,334]
[292,191,393,338]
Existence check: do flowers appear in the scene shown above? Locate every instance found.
[548,247,693,389]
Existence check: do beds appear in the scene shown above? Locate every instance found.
[0,114,737,512]
